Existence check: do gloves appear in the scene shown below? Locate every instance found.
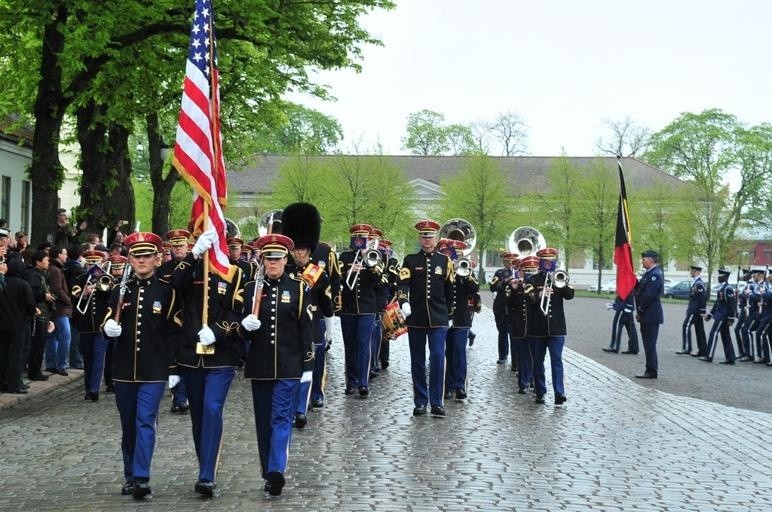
[103,318,122,338]
[605,302,613,311]
[300,370,314,384]
[401,302,413,318]
[197,326,216,346]
[241,313,263,333]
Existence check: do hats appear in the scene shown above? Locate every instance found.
[83,247,127,270]
[347,221,394,257]
[499,246,559,271]
[412,218,470,260]
[688,260,772,284]
[122,232,164,257]
[640,250,659,259]
[166,217,254,265]
[254,234,296,260]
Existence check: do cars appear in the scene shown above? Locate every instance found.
[586,264,772,302]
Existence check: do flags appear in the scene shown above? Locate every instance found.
[172,0,234,284]
[613,154,638,301]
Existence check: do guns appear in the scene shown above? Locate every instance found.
[114,221,140,324]
[251,213,275,319]
[736,264,769,315]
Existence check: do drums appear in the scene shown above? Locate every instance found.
[381,301,408,340]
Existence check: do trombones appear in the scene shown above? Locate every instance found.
[345,238,381,292]
[539,269,569,317]
[452,254,473,277]
[76,261,112,316]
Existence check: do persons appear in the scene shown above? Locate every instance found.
[72,217,342,494]
[601,279,640,355]
[1,209,135,402]
[676,265,772,366]
[489,249,574,405]
[395,221,481,418]
[338,224,400,397]
[633,250,665,379]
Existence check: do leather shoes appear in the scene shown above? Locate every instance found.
[339,360,391,399]
[674,344,771,368]
[84,391,99,402]
[602,344,639,354]
[46,364,70,376]
[262,470,285,496]
[496,356,568,405]
[192,478,218,494]
[27,371,50,382]
[2,380,29,396]
[634,368,658,378]
[294,411,308,428]
[467,333,477,347]
[119,478,151,498]
[170,398,189,412]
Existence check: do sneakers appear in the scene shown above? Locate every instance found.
[411,388,469,418]
